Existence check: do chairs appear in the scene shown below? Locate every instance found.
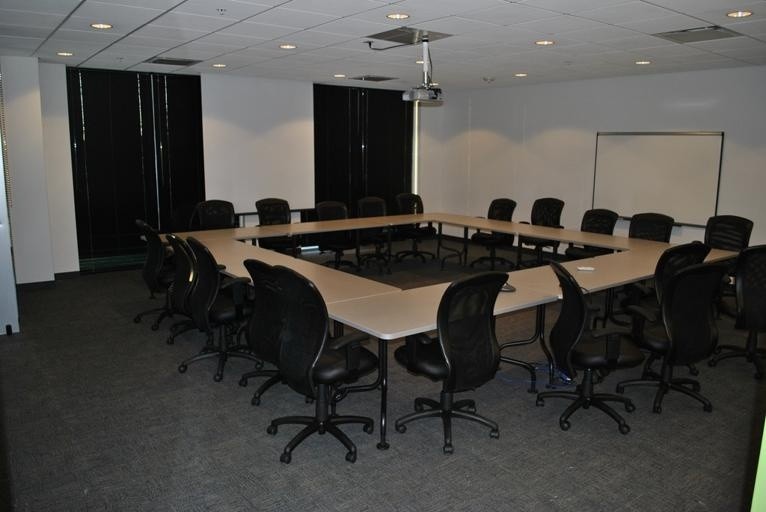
[255,197,293,226]
[239,257,381,463]
[164,231,197,344]
[394,271,510,455]
[134,218,192,330]
[356,196,395,267]
[395,192,437,266]
[704,214,754,251]
[622,242,766,416]
[314,200,355,271]
[469,197,517,271]
[538,255,638,437]
[517,197,566,269]
[190,199,241,230]
[566,208,619,261]
[628,212,675,242]
[177,235,265,381]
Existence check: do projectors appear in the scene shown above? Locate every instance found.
[403,86,447,104]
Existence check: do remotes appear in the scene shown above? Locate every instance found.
[577,265,595,271]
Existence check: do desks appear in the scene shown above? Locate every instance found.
[140,212,740,389]
